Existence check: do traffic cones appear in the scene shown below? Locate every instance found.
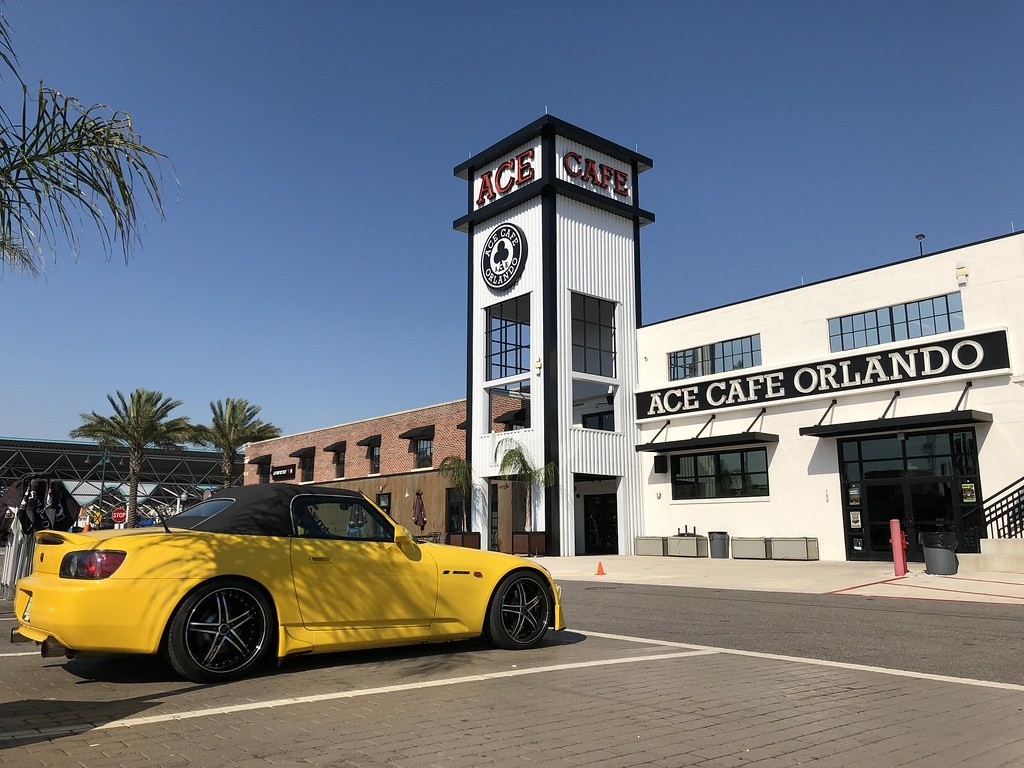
[594,562,608,575]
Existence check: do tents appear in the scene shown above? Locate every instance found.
[0,472,81,598]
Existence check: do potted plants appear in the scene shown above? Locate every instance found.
[494,437,560,556]
[436,454,488,549]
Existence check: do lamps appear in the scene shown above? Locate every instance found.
[404,487,416,498]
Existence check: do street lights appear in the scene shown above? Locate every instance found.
[84,448,126,531]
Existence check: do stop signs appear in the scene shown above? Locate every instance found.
[112,508,127,523]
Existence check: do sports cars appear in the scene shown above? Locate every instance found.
[9,482,567,685]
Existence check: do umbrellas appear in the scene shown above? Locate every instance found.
[350,488,368,538]
[412,490,427,536]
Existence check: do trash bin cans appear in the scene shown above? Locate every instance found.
[708,531,729,559]
[920,531,958,575]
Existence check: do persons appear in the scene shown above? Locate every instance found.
[347,521,366,537]
